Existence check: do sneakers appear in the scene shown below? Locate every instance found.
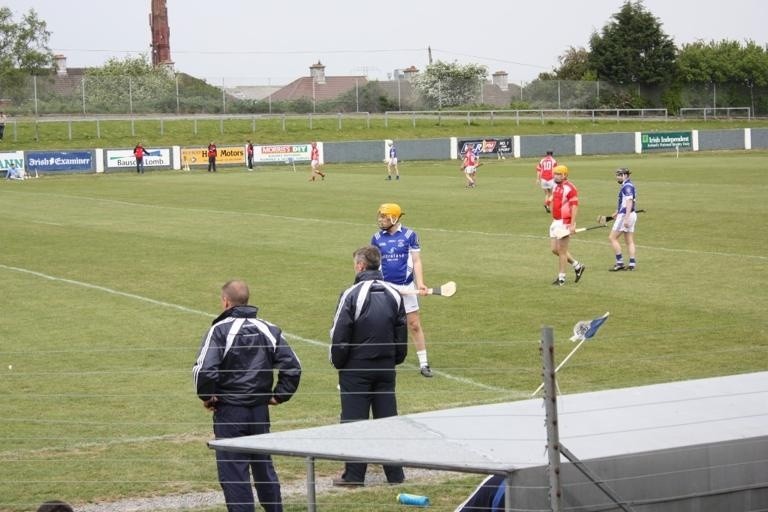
[627,265,635,270]
[544,204,551,213]
[610,262,625,273]
[384,176,400,180]
[333,477,365,486]
[552,278,564,288]
[420,366,433,377]
[574,265,586,282]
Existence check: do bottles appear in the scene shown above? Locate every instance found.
[396,493,428,508]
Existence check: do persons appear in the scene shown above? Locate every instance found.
[134,142,150,174]
[192,280,301,512]
[609,167,637,271]
[370,204,434,377]
[246,140,254,170]
[461,146,477,187]
[550,165,585,286]
[536,151,557,213]
[309,142,326,181]
[37,500,73,512]
[0,112,7,142]
[384,140,399,180]
[4,164,25,180]
[207,141,217,172]
[328,246,407,488]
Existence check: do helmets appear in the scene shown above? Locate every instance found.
[377,204,401,224]
[616,169,630,175]
[553,166,568,176]
